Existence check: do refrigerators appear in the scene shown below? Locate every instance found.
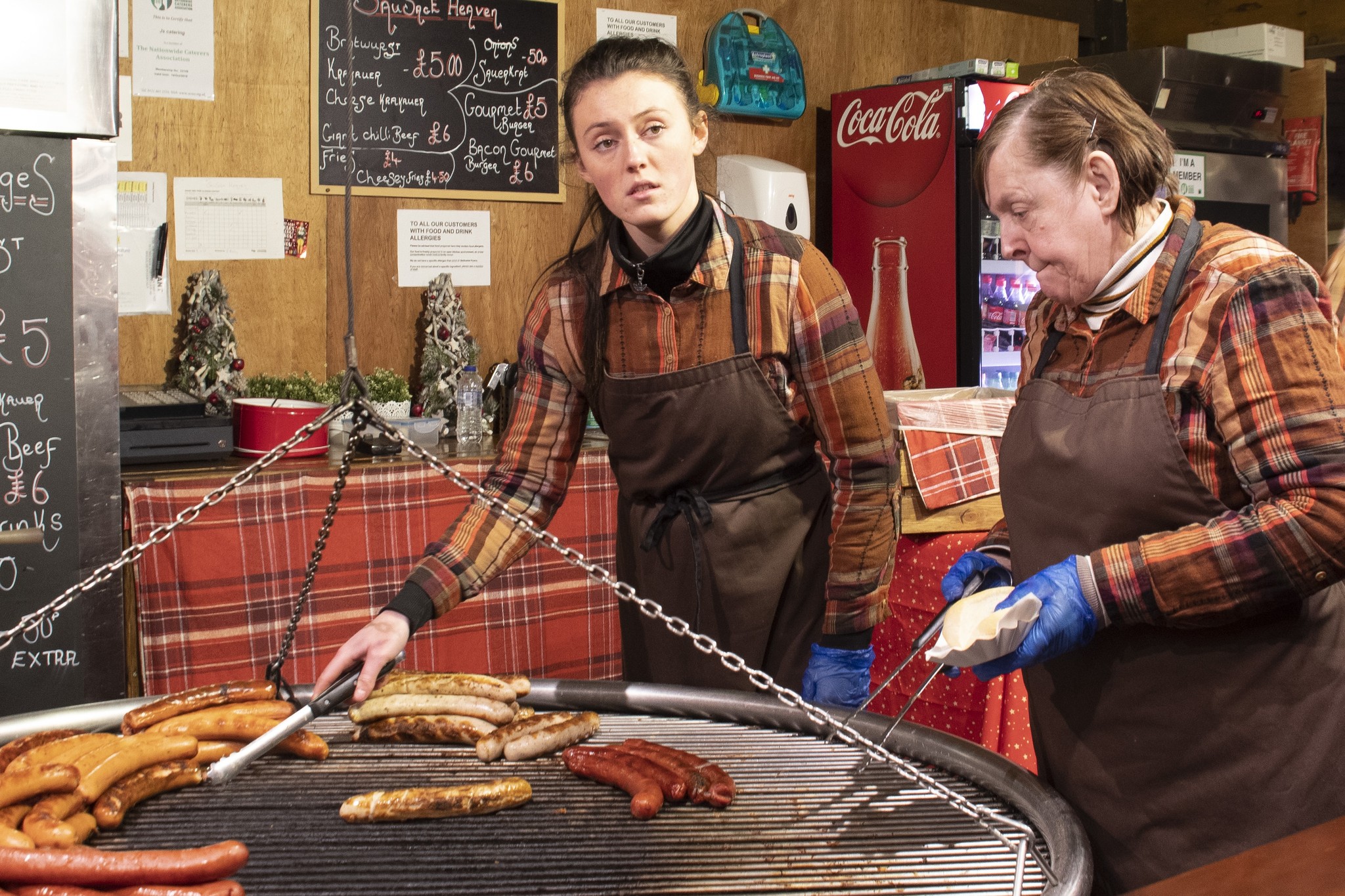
[831,73,1040,390]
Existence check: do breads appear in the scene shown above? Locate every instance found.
[942,586,1017,648]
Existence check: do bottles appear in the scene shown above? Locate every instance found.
[981,373,986,388]
[998,275,1025,351]
[986,372,1004,389]
[866,236,925,391]
[1010,371,1020,390]
[996,370,1012,390]
[988,275,1008,347]
[456,365,483,443]
[981,209,1000,261]
[981,273,993,346]
[1013,279,1038,351]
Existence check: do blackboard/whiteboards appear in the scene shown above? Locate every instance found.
[0,133,83,720]
[308,0,571,202]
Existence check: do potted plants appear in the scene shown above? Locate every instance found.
[248,365,412,426]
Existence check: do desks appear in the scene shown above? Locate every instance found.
[124,438,617,696]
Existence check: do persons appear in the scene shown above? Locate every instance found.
[313,36,904,714]
[942,68,1345,896]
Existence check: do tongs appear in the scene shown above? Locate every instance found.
[207,650,406,786]
[824,564,1015,773]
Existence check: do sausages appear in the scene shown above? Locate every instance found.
[120,678,329,766]
[0,839,249,896]
[563,738,736,820]
[339,777,533,823]
[475,710,600,760]
[0,727,204,848]
[347,668,535,747]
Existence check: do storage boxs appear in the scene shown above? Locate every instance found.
[232,398,329,458]
[1188,23,1304,68]
[342,418,440,450]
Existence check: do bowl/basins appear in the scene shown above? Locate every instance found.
[341,417,440,447]
[232,398,330,458]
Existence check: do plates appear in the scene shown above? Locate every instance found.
[924,586,1042,667]
